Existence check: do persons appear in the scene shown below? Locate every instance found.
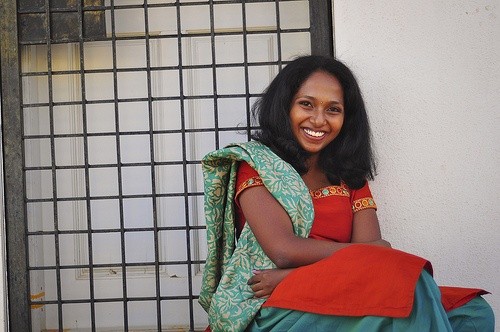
[231,55,496,332]
